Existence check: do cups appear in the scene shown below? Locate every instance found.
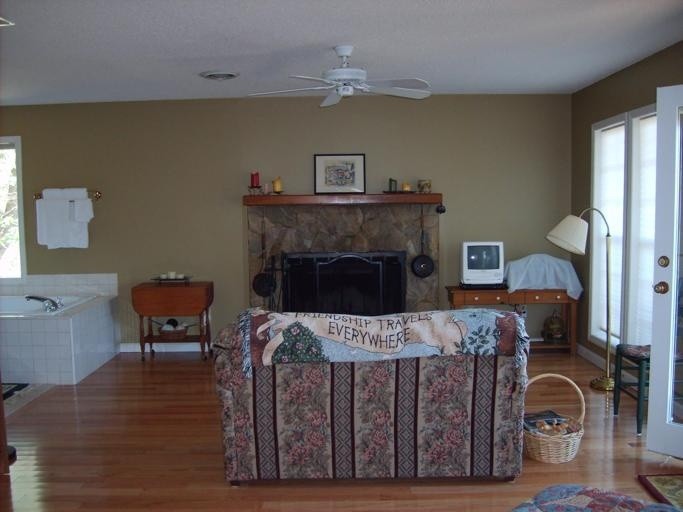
[177,274,184,279]
[168,271,176,279]
[160,274,167,279]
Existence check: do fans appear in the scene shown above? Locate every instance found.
[247,44,432,108]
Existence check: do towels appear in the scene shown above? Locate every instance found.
[73,201,95,223]
[35,198,71,244]
[43,188,89,249]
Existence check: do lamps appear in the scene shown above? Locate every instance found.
[546,206,614,392]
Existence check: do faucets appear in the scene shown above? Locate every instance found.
[26,295,58,311]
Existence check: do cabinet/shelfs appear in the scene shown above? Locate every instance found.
[131,280,214,362]
[445,285,579,356]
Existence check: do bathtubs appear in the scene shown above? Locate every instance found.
[0,292,99,317]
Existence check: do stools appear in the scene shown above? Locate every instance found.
[614,344,683,437]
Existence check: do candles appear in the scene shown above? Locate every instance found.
[401,184,411,191]
[389,178,397,192]
[272,175,281,191]
[250,170,259,186]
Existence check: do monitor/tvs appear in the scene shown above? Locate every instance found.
[458,241,507,289]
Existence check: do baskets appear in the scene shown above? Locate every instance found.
[524,372,586,464]
[159,326,188,341]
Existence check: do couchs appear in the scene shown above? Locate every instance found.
[212,308,530,486]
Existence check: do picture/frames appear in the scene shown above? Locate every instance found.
[314,153,366,195]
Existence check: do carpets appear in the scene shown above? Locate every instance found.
[637,472,683,510]
